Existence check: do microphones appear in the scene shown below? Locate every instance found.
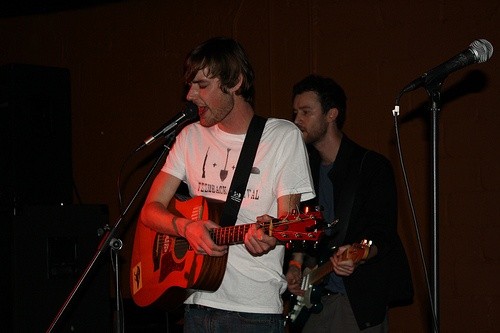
[402,39,493,92]
[131,103,198,152]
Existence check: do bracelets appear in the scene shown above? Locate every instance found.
[289,260,302,269]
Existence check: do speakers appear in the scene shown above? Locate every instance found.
[0,205,113,333]
[0,62,73,206]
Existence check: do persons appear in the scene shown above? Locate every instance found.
[140,36,316,333]
[286,74,414,333]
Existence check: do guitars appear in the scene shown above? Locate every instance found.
[283,239,372,327]
[129,193,340,307]
